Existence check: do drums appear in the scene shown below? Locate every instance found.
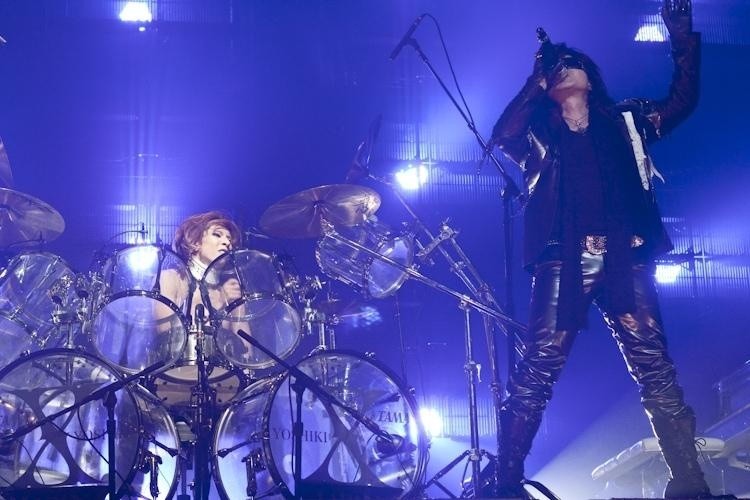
[144,325,247,415]
[0,348,181,500]
[85,244,195,379]
[200,248,305,370]
[211,348,430,500]
[0,249,89,369]
[315,222,417,299]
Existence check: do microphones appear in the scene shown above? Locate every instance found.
[536,26,568,79]
[141,222,145,240]
[388,14,423,62]
[194,303,204,340]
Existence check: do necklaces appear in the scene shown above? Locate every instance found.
[562,110,591,136]
[187,253,225,287]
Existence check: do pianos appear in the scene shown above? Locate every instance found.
[591,437,724,481]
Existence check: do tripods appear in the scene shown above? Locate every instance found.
[403,304,560,500]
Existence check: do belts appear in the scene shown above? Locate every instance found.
[584,233,644,255]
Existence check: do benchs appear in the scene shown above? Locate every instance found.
[591,437,726,497]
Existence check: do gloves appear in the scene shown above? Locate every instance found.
[533,40,570,92]
[659,1,693,44]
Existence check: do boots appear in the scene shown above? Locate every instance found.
[651,402,731,498]
[493,405,543,499]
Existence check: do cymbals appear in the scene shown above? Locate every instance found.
[259,184,381,239]
[1,187,66,249]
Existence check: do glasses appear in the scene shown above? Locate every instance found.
[562,56,583,70]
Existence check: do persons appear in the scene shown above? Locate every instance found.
[148,210,254,377]
[481,1,708,499]
